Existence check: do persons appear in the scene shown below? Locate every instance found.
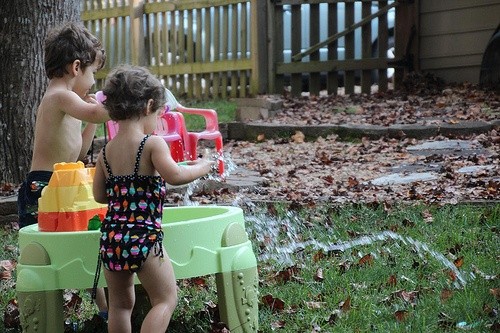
[18,23,111,317]
[93,63,215,333]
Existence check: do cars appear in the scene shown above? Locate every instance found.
[84,0,397,99]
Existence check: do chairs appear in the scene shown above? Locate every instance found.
[163,87,224,179]
[92,90,185,162]
